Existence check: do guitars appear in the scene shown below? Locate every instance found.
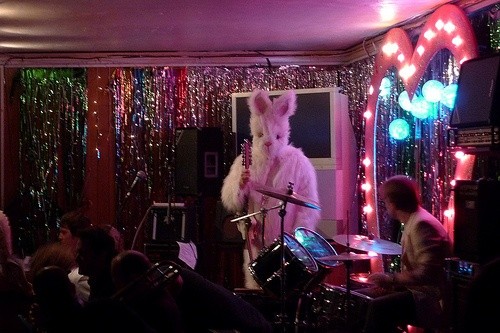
[232,138,258,241]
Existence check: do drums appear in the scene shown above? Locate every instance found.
[235,287,297,333]
[247,230,318,303]
[293,226,339,292]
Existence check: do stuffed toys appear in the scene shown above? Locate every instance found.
[222,87,323,288]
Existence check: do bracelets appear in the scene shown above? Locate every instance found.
[392,278,395,283]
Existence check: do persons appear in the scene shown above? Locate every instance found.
[368,176,451,333]
[0,210,274,333]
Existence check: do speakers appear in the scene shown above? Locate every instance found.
[174,127,225,198]
[450,53,500,127]
[444,257,500,333]
[453,179,500,259]
[143,242,203,274]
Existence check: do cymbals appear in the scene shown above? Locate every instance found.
[332,235,402,254]
[313,252,378,260]
[256,189,321,210]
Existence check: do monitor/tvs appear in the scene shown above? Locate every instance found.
[231,88,339,166]
